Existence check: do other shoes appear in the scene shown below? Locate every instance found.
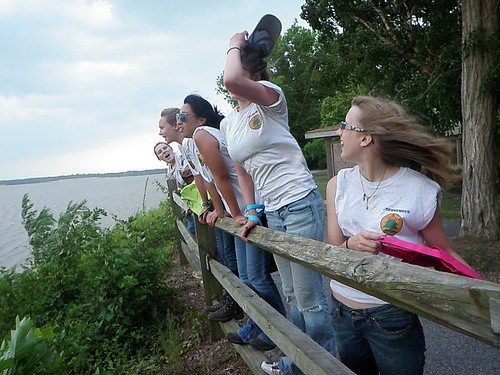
[193,272,203,284]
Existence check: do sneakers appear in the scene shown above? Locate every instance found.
[260,360,287,375]
[208,296,244,324]
[249,337,277,351]
[204,292,229,313]
[225,332,250,346]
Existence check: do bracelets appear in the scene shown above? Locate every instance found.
[227,46,241,54]
[346,237,349,248]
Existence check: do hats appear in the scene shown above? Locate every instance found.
[234,14,282,76]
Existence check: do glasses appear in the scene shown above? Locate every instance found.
[340,121,376,145]
[176,112,194,122]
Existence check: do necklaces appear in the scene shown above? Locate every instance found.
[361,165,386,209]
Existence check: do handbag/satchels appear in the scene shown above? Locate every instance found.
[179,180,212,217]
[370,235,486,280]
[257,212,279,272]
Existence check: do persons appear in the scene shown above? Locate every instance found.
[326,96,474,375]
[153,108,244,321]
[176,95,286,349]
[221,30,336,375]
